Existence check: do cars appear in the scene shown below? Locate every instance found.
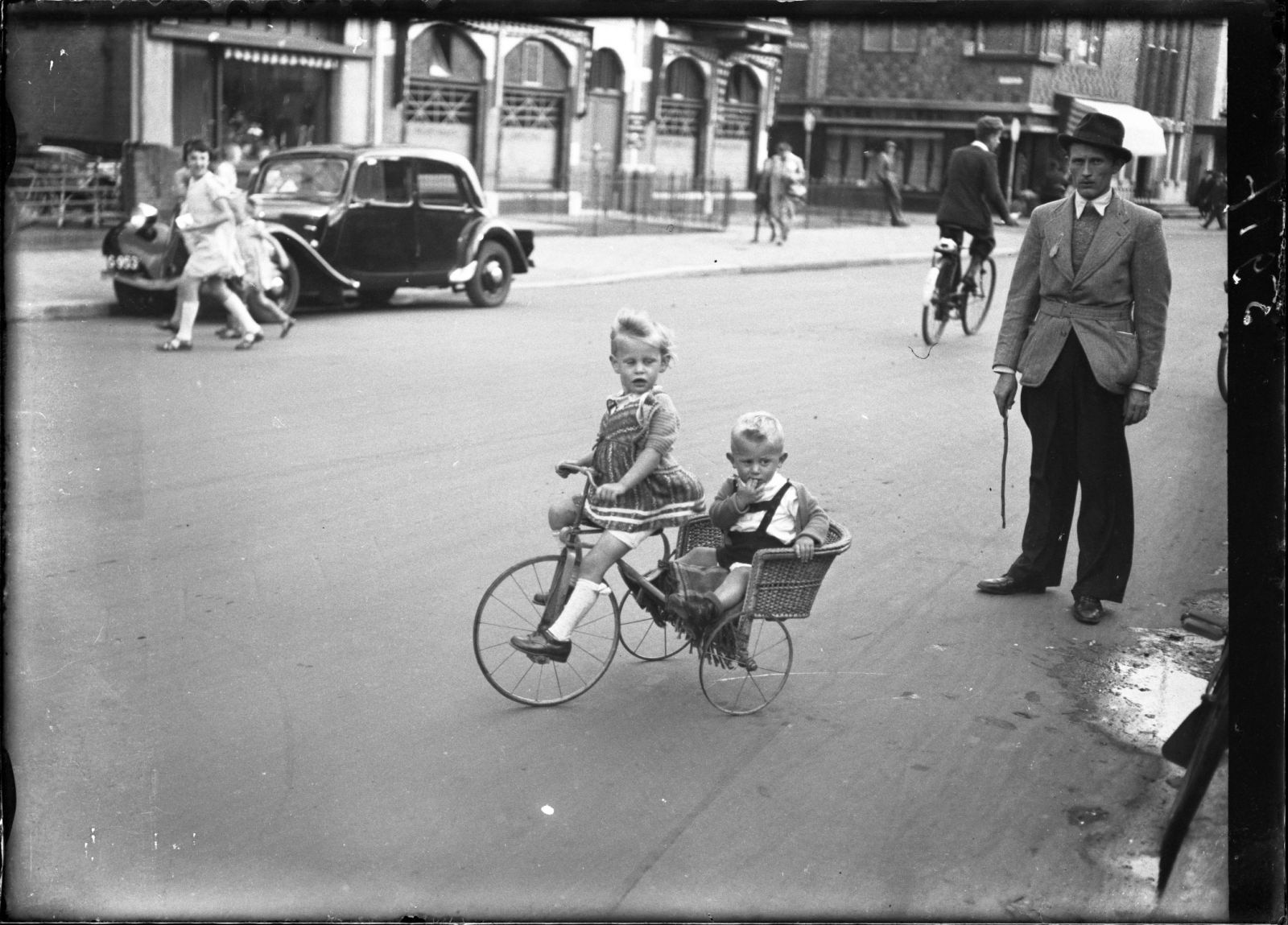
[101,144,535,322]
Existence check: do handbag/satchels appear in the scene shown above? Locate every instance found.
[787,182,807,198]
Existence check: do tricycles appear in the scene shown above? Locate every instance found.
[473,464,852,715]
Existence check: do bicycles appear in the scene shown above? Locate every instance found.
[922,221,1018,345]
[1218,279,1228,402]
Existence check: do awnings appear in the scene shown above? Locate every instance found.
[1067,97,1167,158]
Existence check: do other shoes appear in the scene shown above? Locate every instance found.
[215,325,232,333]
[931,294,942,309]
[280,317,296,338]
[235,328,262,351]
[961,274,978,290]
[155,335,193,352]
[220,328,239,339]
[670,595,712,643]
[155,320,178,333]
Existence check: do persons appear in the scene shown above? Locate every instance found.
[1194,170,1227,230]
[978,112,1171,623]
[935,116,1018,321]
[764,142,806,246]
[156,140,297,351]
[875,140,909,227]
[751,159,776,243]
[510,311,706,663]
[618,412,828,639]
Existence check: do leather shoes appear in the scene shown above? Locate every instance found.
[509,628,570,664]
[535,586,574,607]
[1072,595,1103,625]
[977,572,1048,595]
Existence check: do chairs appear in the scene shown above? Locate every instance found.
[385,162,409,203]
[676,509,851,649]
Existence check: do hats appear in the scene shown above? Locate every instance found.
[1059,111,1132,164]
[976,116,1003,131]
